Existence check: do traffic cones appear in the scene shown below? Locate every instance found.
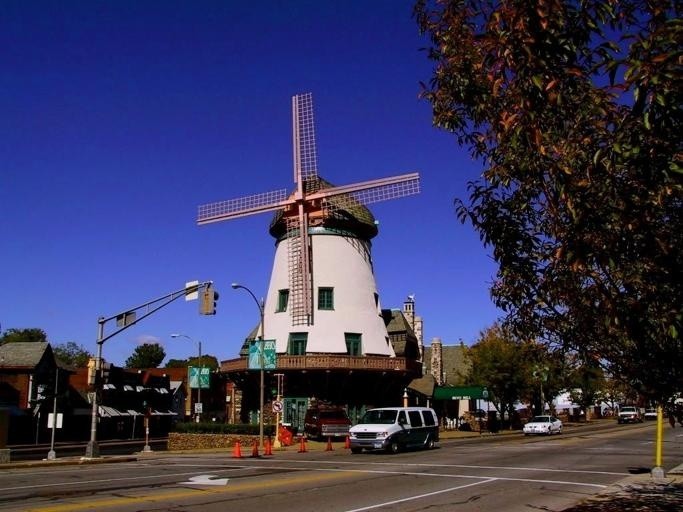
[233,441,241,458]
[297,439,305,453]
[249,440,260,458]
[344,437,350,449]
[325,437,332,451]
[263,437,272,456]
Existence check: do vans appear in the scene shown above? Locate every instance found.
[347,407,439,454]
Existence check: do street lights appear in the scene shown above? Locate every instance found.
[231,282,267,457]
[170,334,201,424]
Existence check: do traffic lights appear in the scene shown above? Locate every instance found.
[213,291,218,315]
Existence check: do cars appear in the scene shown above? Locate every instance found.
[522,415,562,436]
[617,405,645,423]
[304,408,351,442]
[644,409,657,420]
